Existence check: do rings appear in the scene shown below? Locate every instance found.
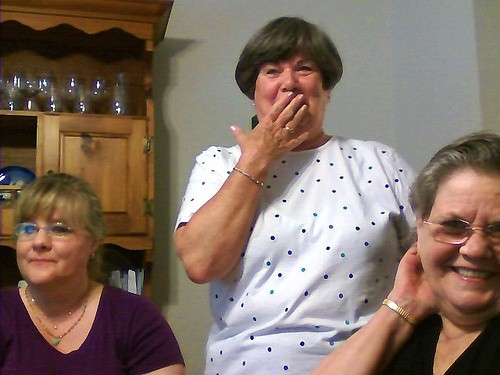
[285,127,293,135]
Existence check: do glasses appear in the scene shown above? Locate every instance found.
[423,217,500,245]
[14,223,88,241]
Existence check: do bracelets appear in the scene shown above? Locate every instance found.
[233,167,264,187]
[382,299,416,325]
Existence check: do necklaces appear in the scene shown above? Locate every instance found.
[25,286,88,344]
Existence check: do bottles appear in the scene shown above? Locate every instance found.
[48,84,59,113]
[111,74,127,115]
[0,74,37,111]
[93,79,104,97]
[65,78,77,95]
[77,85,88,113]
[38,75,51,94]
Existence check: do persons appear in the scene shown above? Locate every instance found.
[312,131,500,375]
[174,16,419,375]
[0,173,186,375]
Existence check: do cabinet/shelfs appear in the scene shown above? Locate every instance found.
[0,0,174,296]
